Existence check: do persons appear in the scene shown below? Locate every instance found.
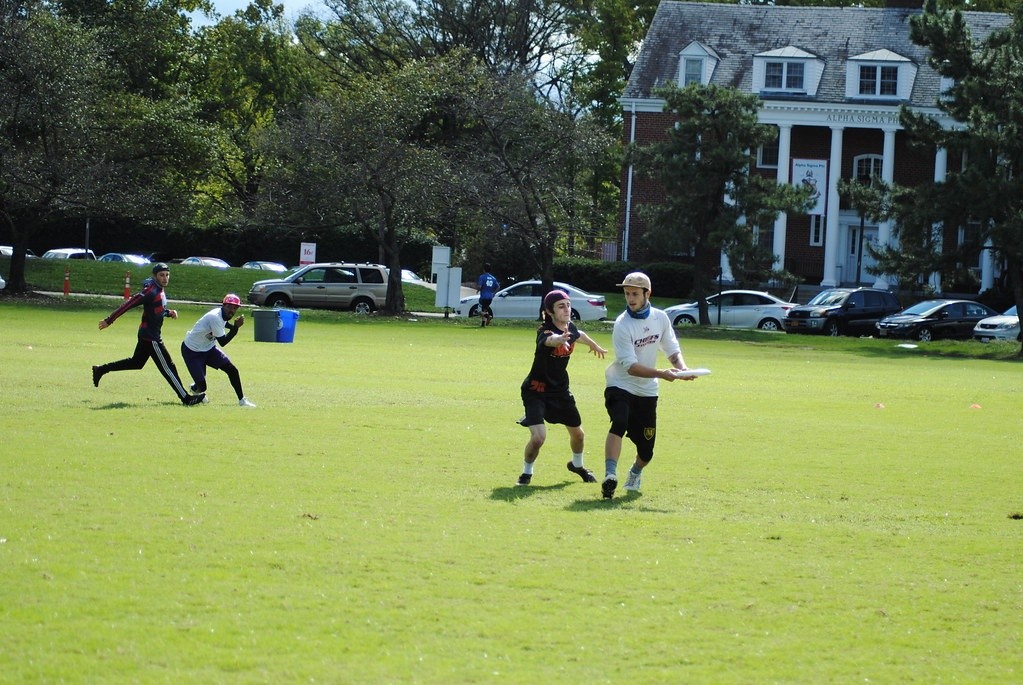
[515,290,607,485]
[476,263,499,326]
[91,262,205,407]
[601,271,698,500]
[181,294,257,407]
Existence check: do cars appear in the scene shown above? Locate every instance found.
[454,280,607,321]
[385,268,423,280]
[97,252,152,263]
[0,246,35,258]
[180,256,231,267]
[972,304,1022,343]
[663,289,801,331]
[240,260,288,271]
[42,247,97,260]
[875,298,1001,342]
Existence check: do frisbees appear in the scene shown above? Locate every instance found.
[676,368,712,378]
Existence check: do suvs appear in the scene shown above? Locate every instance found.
[783,286,905,338]
[247,260,389,315]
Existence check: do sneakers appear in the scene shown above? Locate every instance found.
[486,315,492,326]
[623,470,641,492]
[480,326,486,328]
[92,365,104,387]
[601,474,617,499]
[239,397,255,407]
[183,393,205,405]
[567,460,598,483]
[190,384,209,403]
[517,473,532,486]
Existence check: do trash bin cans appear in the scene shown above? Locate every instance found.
[251,309,281,342]
[278,309,300,343]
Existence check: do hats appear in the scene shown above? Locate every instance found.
[223,294,241,307]
[616,271,652,292]
[153,263,170,274]
[542,290,570,309]
